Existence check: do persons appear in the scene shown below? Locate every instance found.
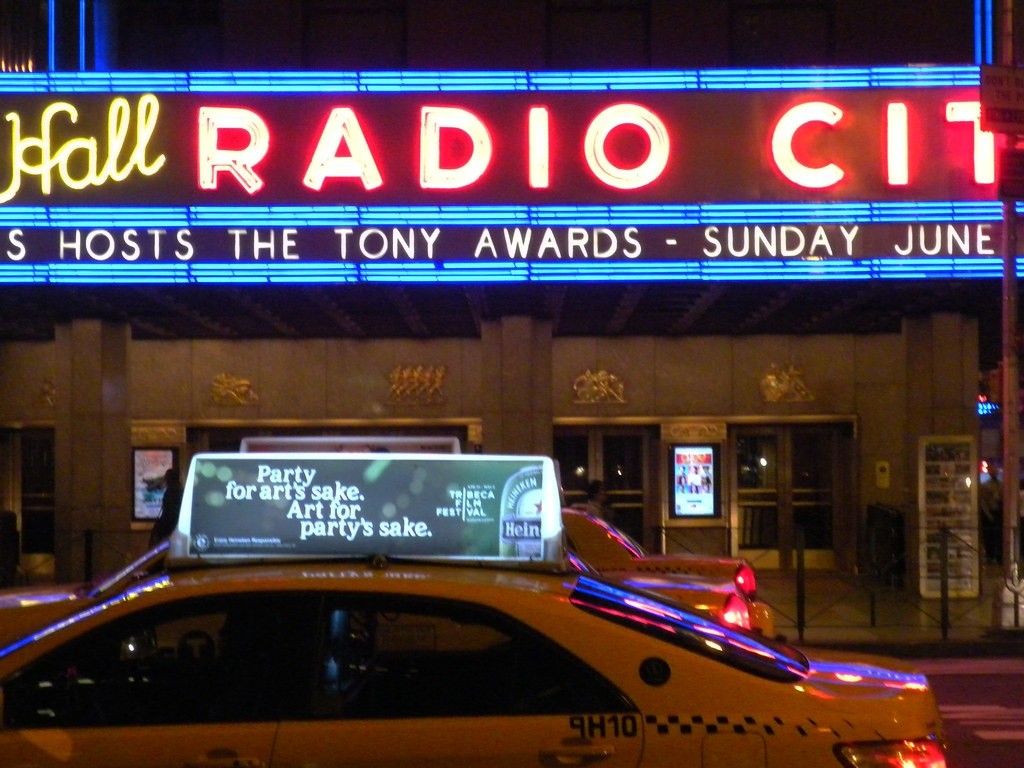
[980,463,1004,566]
[582,479,606,521]
[676,465,712,494]
[167,610,275,724]
[145,467,185,548]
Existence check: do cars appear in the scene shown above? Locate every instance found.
[0,452,947,768]
[0,438,780,640]
